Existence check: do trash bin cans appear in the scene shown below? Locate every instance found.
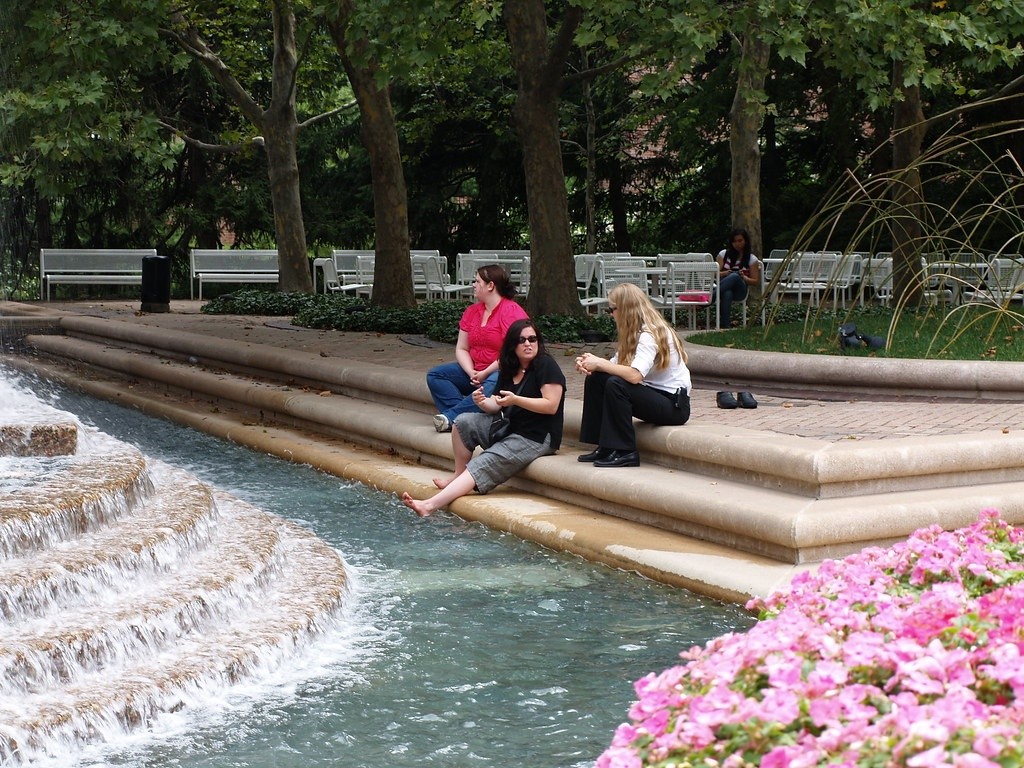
[140,254,174,313]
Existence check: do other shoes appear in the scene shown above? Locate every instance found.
[433,414,449,432]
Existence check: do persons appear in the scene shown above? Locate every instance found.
[426,264,528,432]
[696,229,760,329]
[573,283,692,468]
[403,319,566,517]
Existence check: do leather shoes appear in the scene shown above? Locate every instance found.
[594,448,640,466]
[577,445,613,461]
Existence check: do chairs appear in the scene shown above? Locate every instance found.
[520,256,531,300]
[412,255,474,301]
[600,261,649,300]
[731,259,767,329]
[314,258,372,301]
[577,292,610,314]
[650,262,720,332]
[687,251,720,331]
[594,252,632,314]
[766,247,1024,310]
[572,253,604,312]
[455,253,499,302]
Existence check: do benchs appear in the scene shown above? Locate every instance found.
[468,248,531,298]
[355,256,448,301]
[39,248,157,302]
[332,249,451,301]
[189,249,279,301]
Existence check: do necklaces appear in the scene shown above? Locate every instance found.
[519,368,526,373]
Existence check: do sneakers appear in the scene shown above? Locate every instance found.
[737,392,757,409]
[716,391,738,408]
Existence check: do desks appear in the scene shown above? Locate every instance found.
[614,266,698,305]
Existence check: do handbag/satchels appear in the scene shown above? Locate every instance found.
[680,290,709,301]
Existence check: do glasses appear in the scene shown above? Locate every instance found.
[519,335,537,344]
[605,306,617,314]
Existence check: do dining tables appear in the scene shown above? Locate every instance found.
[759,258,802,304]
[473,253,523,299]
[611,256,688,308]
[927,263,997,306]
